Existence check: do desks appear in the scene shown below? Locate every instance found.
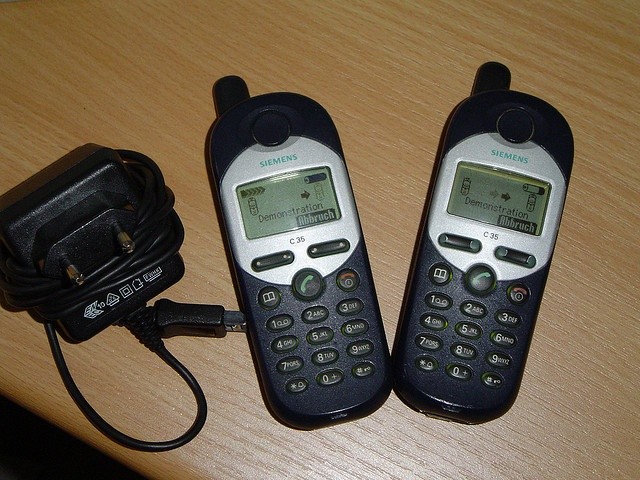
[0,0,638,480]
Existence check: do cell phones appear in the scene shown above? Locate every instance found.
[208,76,393,431]
[393,61,574,425]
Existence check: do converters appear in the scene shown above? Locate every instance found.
[0,142,185,344]
